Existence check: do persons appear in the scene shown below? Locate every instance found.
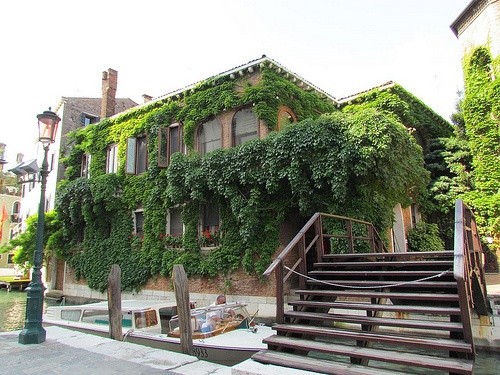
[202,293,237,335]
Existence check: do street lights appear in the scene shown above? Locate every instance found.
[7,106,62,343]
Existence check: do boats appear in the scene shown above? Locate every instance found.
[40,298,279,367]
